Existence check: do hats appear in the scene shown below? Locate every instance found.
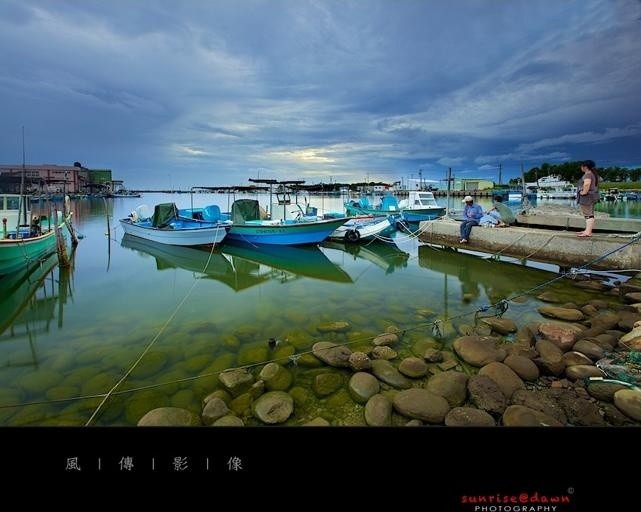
[462,196,472,202]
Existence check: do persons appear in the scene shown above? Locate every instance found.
[574,159,604,238]
[459,195,484,244]
[30,216,45,238]
[486,195,516,228]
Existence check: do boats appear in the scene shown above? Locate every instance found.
[322,236,409,275]
[0,246,72,337]
[115,235,254,282]
[0,189,77,277]
[110,185,141,198]
[224,243,360,293]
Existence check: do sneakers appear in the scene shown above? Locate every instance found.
[496,225,509,228]
[576,231,592,237]
